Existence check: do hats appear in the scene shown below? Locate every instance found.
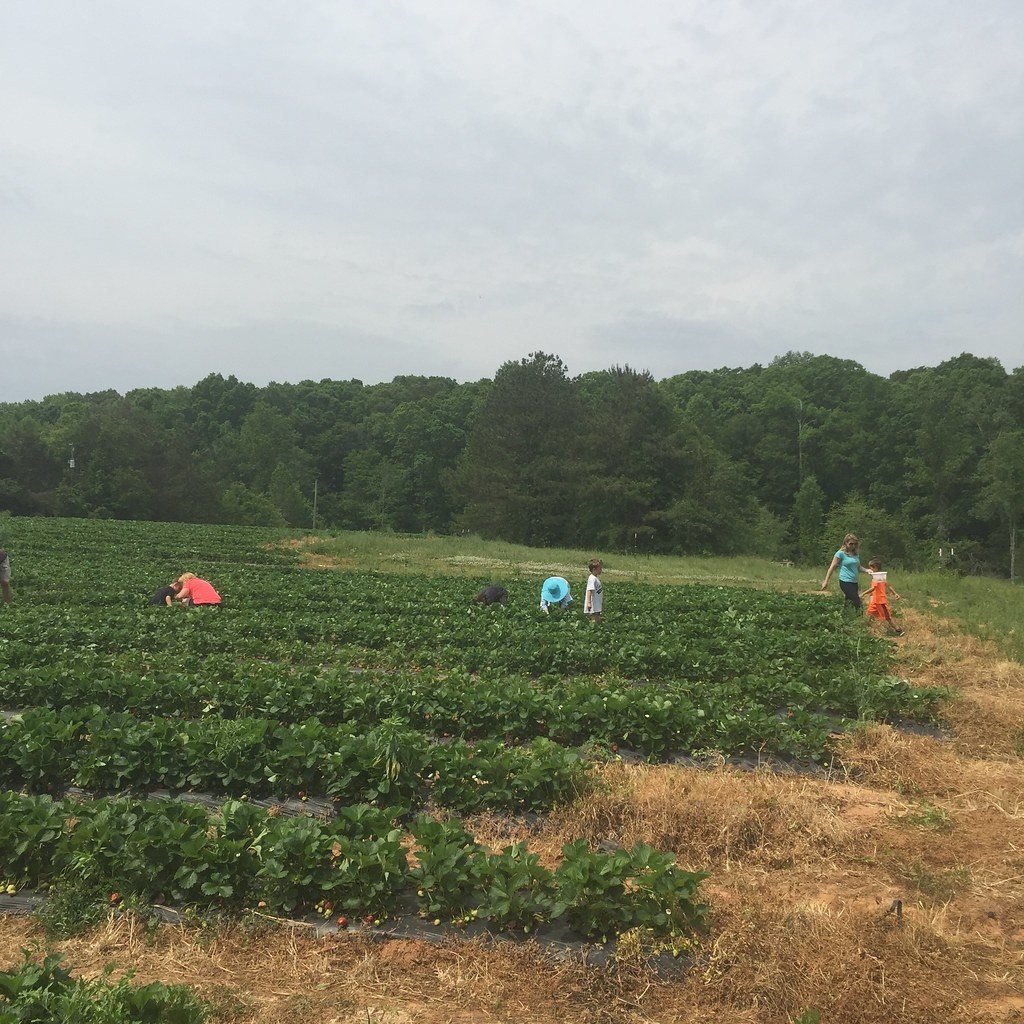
[541,577,568,602]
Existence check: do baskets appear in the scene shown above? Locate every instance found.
[869,572,887,583]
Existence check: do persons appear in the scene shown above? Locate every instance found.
[540,576,573,615]
[0,549,12,605]
[147,578,182,607]
[473,585,508,606]
[858,558,905,637]
[175,572,223,610]
[822,533,873,616]
[584,558,603,624]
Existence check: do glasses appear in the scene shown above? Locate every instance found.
[848,542,858,546]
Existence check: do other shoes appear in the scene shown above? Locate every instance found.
[895,629,902,636]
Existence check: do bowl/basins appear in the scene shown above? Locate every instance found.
[871,572,887,582]
